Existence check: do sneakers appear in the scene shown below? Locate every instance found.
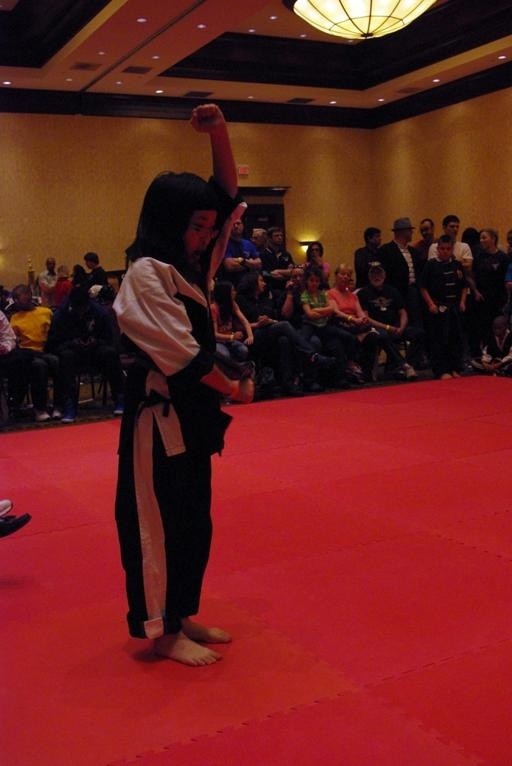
[113,404,123,415]
[53,410,63,419]
[35,411,51,421]
[405,365,418,380]
[61,415,75,422]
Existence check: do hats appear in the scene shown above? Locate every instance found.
[391,217,415,232]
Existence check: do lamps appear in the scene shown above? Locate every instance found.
[282,0,437,40]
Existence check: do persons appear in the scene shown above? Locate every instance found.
[110,103,254,668]
[206,215,512,405]
[1,252,138,423]
